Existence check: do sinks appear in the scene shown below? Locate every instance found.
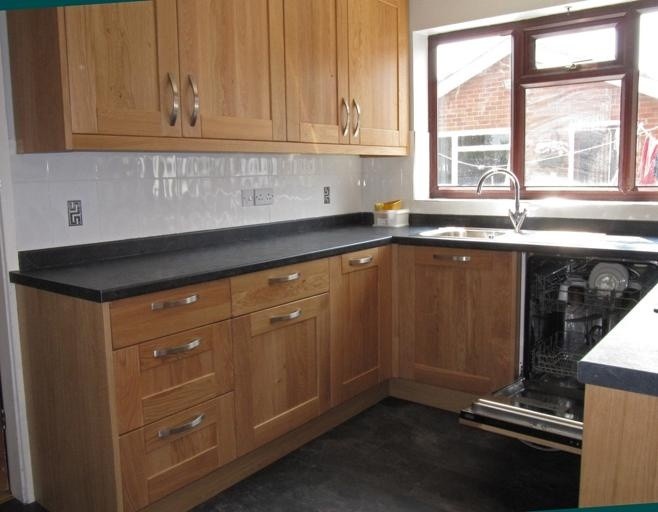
[413,225,504,239]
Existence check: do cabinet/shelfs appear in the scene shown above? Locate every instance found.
[394,246,519,416]
[282,0,413,159]
[7,0,286,156]
[331,241,395,433]
[234,255,331,481]
[17,277,234,509]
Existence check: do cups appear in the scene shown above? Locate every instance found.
[374,202,384,211]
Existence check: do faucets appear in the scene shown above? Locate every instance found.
[477,167,527,231]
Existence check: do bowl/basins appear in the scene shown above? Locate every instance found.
[557,262,646,301]
[383,200,400,210]
